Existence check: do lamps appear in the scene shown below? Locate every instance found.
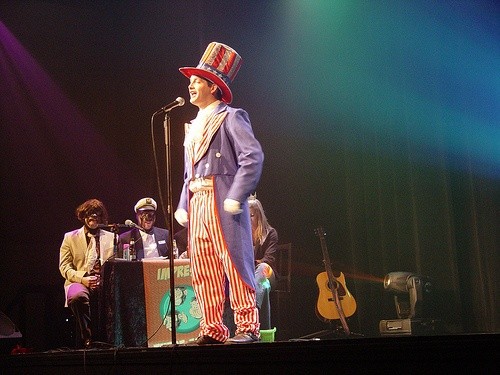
[379,271,431,334]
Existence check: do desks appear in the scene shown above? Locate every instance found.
[103,259,201,347]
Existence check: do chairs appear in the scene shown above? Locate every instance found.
[272,243,292,340]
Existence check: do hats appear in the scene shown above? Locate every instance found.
[179,41,243,105]
[134,197,158,213]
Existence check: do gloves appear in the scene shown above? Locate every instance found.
[174,209,190,227]
[223,199,243,216]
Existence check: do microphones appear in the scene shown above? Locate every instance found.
[152,98,185,116]
[125,219,147,232]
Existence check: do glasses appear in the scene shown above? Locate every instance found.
[250,212,256,217]
[91,213,101,218]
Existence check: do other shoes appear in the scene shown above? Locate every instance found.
[187,336,224,345]
[84,339,91,348]
[225,332,257,344]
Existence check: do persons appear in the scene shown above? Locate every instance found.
[247,196,278,328]
[58,198,119,350]
[174,228,188,260]
[117,198,169,260]
[174,42,264,344]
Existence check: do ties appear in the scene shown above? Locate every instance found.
[85,232,97,274]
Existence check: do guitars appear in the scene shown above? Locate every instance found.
[313,226,359,324]
